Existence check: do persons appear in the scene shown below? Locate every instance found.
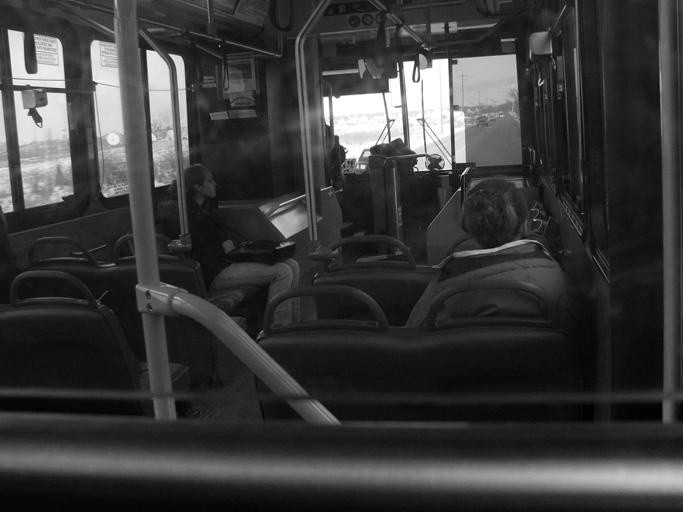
[404,176,578,327]
[166,163,300,329]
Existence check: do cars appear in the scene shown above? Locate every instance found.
[488,112,498,121]
[476,116,488,127]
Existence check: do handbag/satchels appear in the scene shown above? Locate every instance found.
[228,239,297,266]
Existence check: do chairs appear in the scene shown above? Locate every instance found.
[0,223,594,426]
